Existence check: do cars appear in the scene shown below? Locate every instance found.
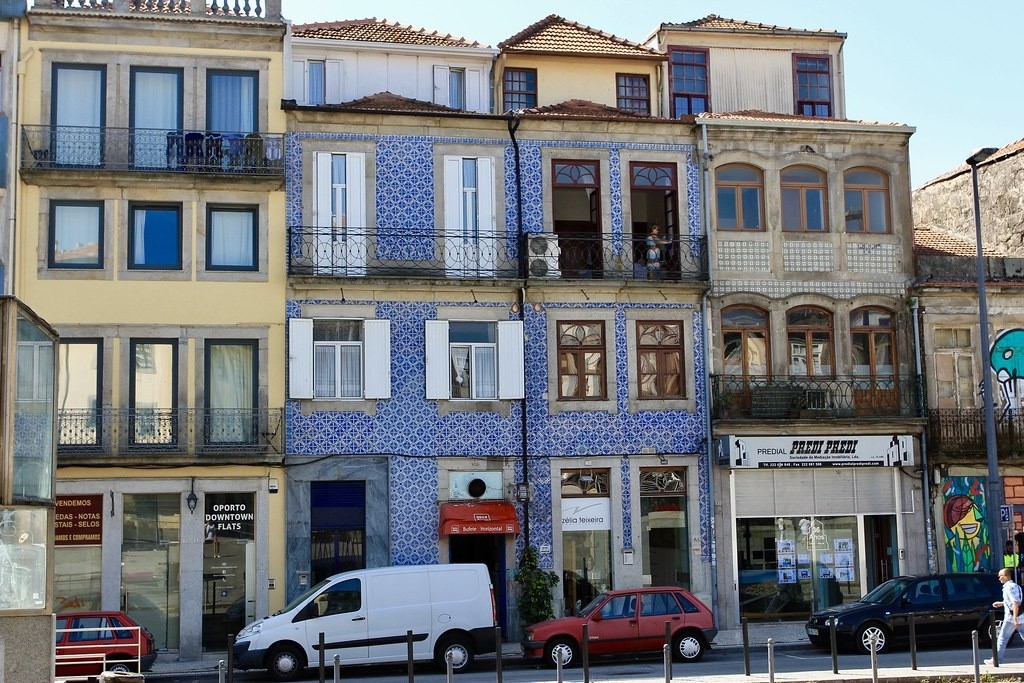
[805,570,1004,653]
[56,611,157,678]
[520,585,718,669]
[737,564,844,620]
[53,589,169,648]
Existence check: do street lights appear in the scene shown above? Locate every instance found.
[966,146,1006,570]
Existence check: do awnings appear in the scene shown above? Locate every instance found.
[439,501,520,539]
[714,432,915,469]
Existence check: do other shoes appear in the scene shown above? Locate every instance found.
[984,657,994,665]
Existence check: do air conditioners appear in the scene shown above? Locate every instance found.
[527,233,559,256]
[528,257,559,277]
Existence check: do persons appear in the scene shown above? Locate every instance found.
[323,590,352,617]
[646,223,672,280]
[1003,541,1020,587]
[984,569,1024,666]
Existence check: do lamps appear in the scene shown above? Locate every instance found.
[186,476,199,514]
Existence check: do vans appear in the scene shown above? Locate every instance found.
[225,554,363,647]
[233,563,498,683]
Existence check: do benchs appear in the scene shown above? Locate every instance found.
[950,583,992,600]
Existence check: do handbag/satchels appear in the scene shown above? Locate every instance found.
[1005,586,1024,617]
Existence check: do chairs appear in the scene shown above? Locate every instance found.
[626,598,644,617]
[931,586,940,602]
[78,624,85,640]
[915,585,933,602]
